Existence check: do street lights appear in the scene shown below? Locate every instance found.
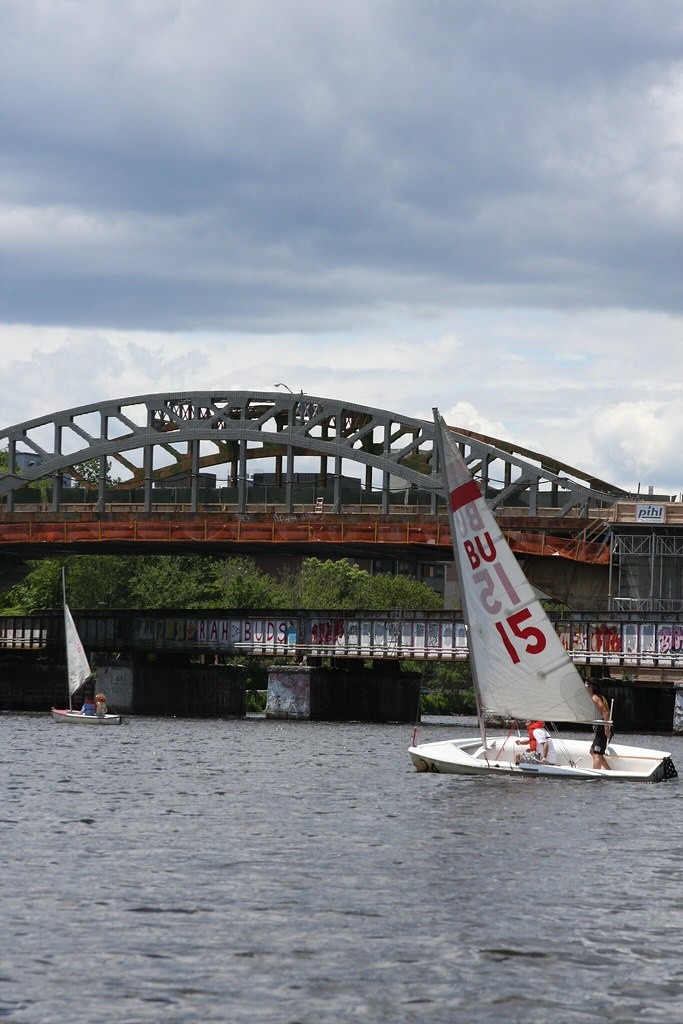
[275,383,293,394]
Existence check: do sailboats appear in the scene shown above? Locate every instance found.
[407,406,677,785]
[50,565,124,726]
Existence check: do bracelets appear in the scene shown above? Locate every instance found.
[543,757,546,760]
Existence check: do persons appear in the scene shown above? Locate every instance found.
[585,680,615,770]
[78,693,107,716]
[515,720,557,766]
